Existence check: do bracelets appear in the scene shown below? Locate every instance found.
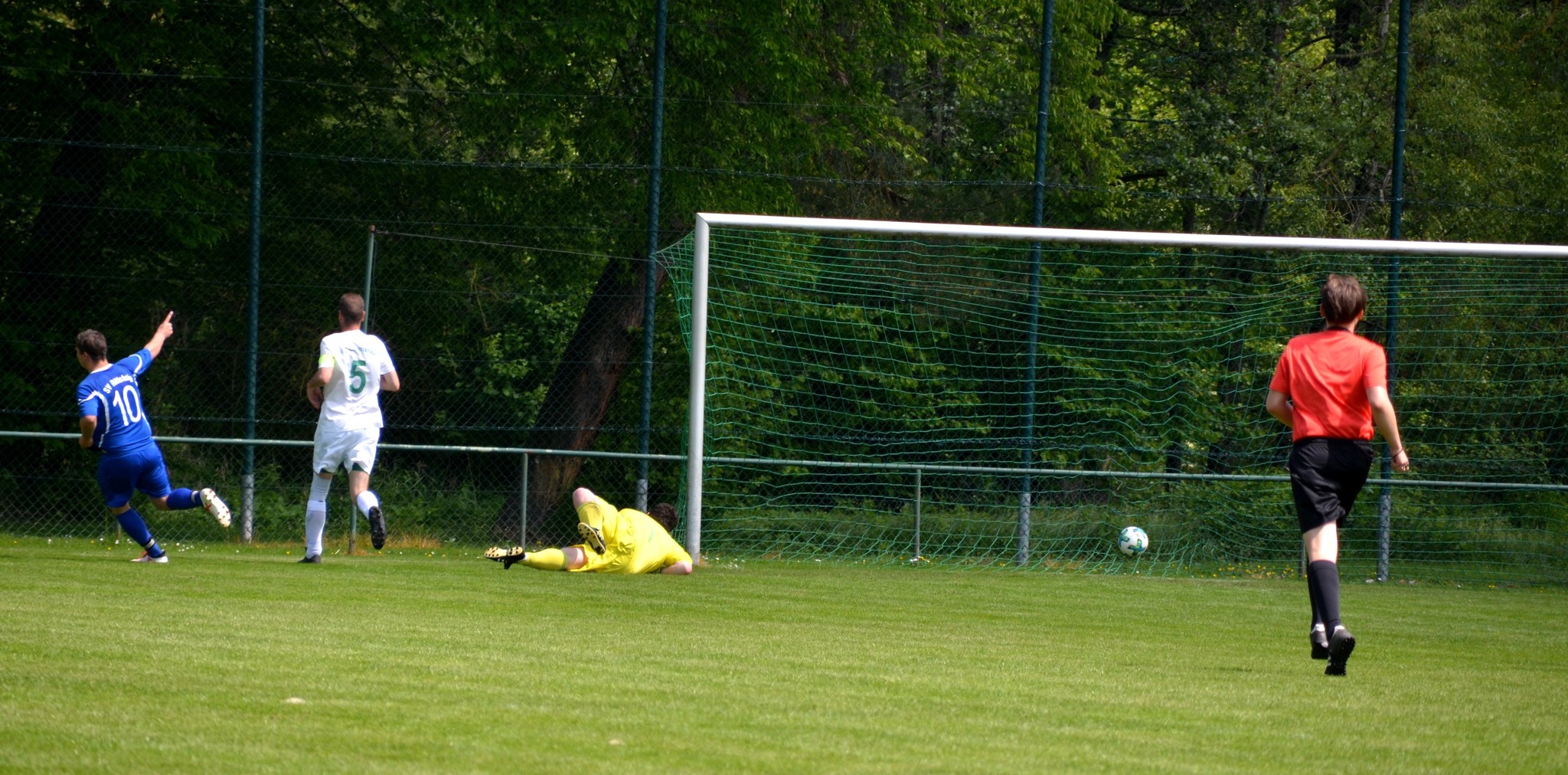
[1391,449,1403,459]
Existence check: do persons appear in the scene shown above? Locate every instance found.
[296,293,399,563]
[485,488,693,575]
[75,312,230,563]
[1267,273,1410,677]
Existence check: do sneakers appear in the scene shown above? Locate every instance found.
[130,549,169,564]
[577,522,607,555]
[369,506,387,550]
[295,555,321,564]
[483,546,526,569]
[1309,623,1329,660]
[1324,625,1356,676]
[199,487,233,527]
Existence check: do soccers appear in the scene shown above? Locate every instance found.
[1118,526,1149,558]
